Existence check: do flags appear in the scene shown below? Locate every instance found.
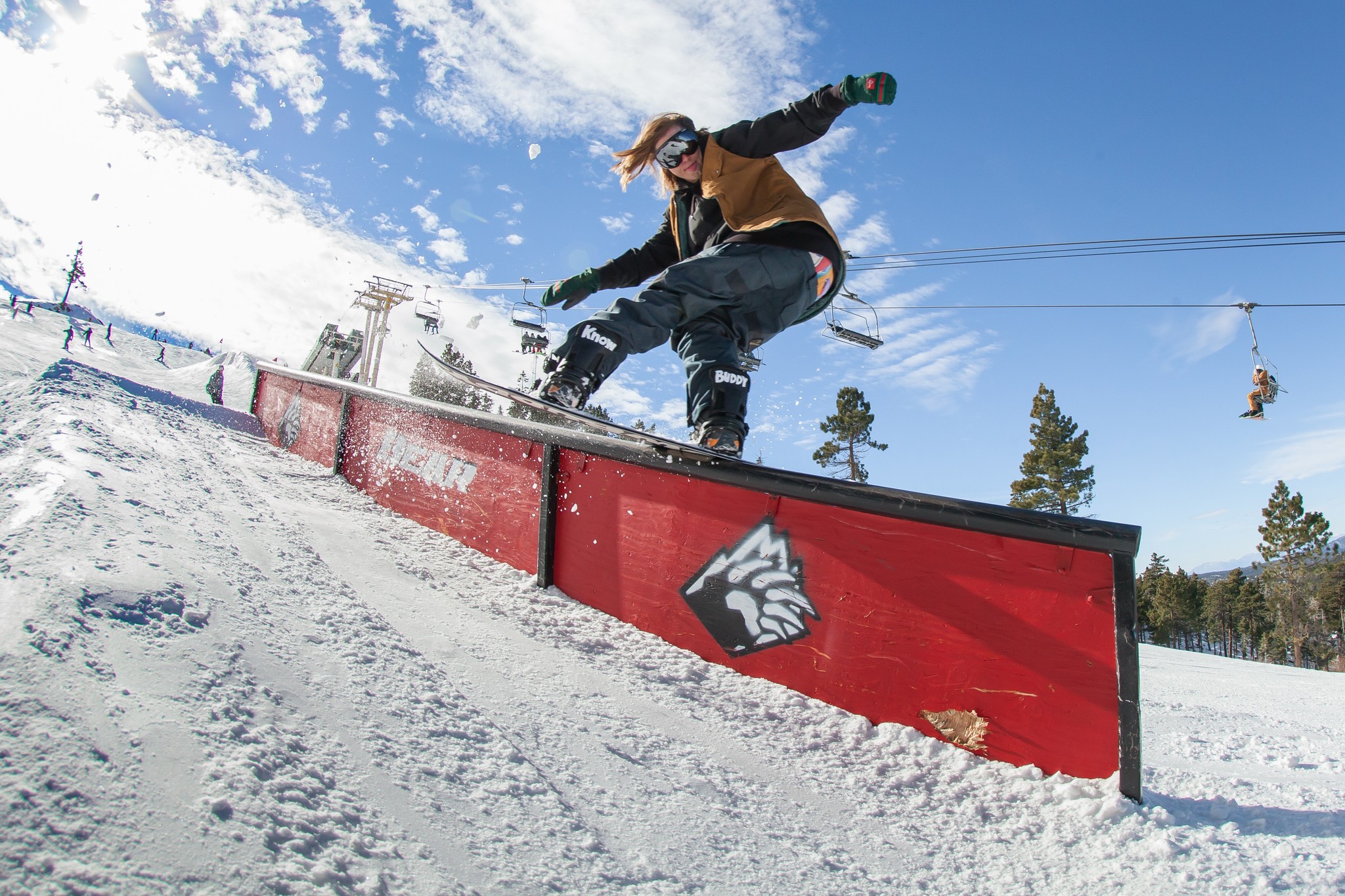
[220,339,222,343]
[273,357,277,362]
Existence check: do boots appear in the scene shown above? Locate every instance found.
[528,322,622,411]
[687,368,752,459]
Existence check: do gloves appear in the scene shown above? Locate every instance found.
[540,266,600,311]
[840,72,897,106]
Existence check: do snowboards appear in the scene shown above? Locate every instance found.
[416,338,770,472]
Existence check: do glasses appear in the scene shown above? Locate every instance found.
[654,127,699,169]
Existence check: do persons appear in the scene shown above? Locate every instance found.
[88,317,92,322]
[1246,365,1275,417]
[528,333,536,354]
[188,342,193,349]
[536,334,548,356]
[61,326,73,351]
[209,365,224,406]
[539,71,897,460]
[206,348,210,354]
[425,324,438,334]
[521,331,529,354]
[157,347,165,362]
[104,323,112,341]
[9,294,19,307]
[152,329,158,341]
[28,301,35,313]
[83,328,93,347]
